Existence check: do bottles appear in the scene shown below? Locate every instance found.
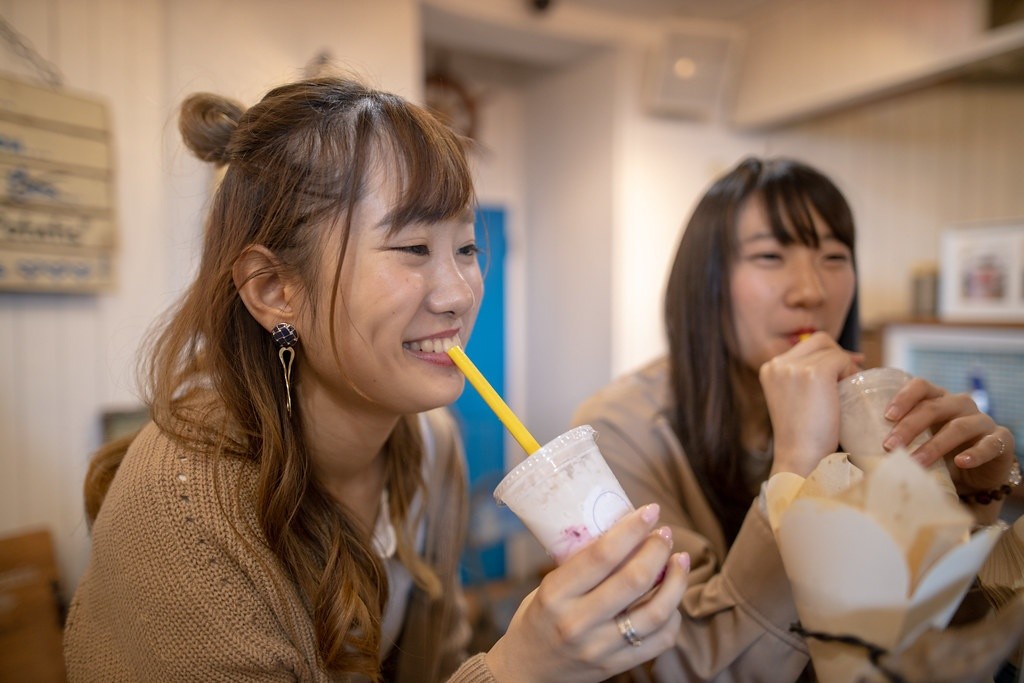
[971,379,989,413]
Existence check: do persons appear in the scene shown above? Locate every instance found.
[64,74,692,683]
[576,154,1024,683]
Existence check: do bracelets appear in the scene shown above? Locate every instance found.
[956,459,1023,506]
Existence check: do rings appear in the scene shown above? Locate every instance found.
[997,438,1005,455]
[614,610,641,645]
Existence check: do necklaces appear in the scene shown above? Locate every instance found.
[745,445,774,462]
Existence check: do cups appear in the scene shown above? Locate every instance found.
[492,425,669,617]
[913,260,935,316]
[838,367,960,502]
